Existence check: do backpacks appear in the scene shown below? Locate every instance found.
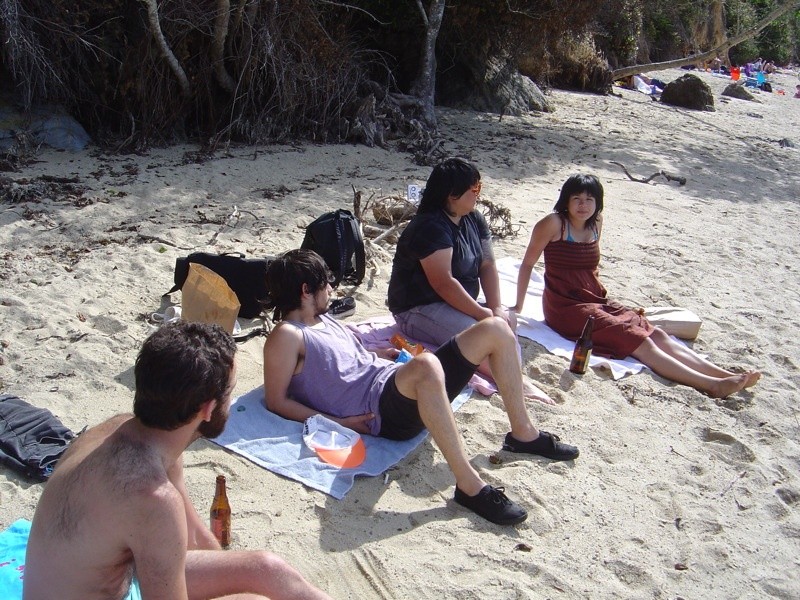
[761,82,772,93]
[302,209,365,287]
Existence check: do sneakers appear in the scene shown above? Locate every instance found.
[454,482,528,525]
[503,430,579,460]
[327,297,355,318]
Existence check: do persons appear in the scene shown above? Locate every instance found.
[636,73,667,90]
[506,174,762,399]
[387,158,557,406]
[21,318,331,600]
[754,57,777,74]
[255,249,580,527]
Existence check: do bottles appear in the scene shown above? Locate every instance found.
[570,314,596,375]
[209,475,232,549]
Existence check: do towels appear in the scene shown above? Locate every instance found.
[203,383,470,501]
[476,254,711,381]
[0,517,32,600]
[342,316,521,398]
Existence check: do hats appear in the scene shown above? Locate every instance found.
[303,414,365,469]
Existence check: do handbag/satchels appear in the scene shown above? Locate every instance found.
[174,250,273,318]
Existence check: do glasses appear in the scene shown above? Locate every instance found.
[465,182,481,195]
